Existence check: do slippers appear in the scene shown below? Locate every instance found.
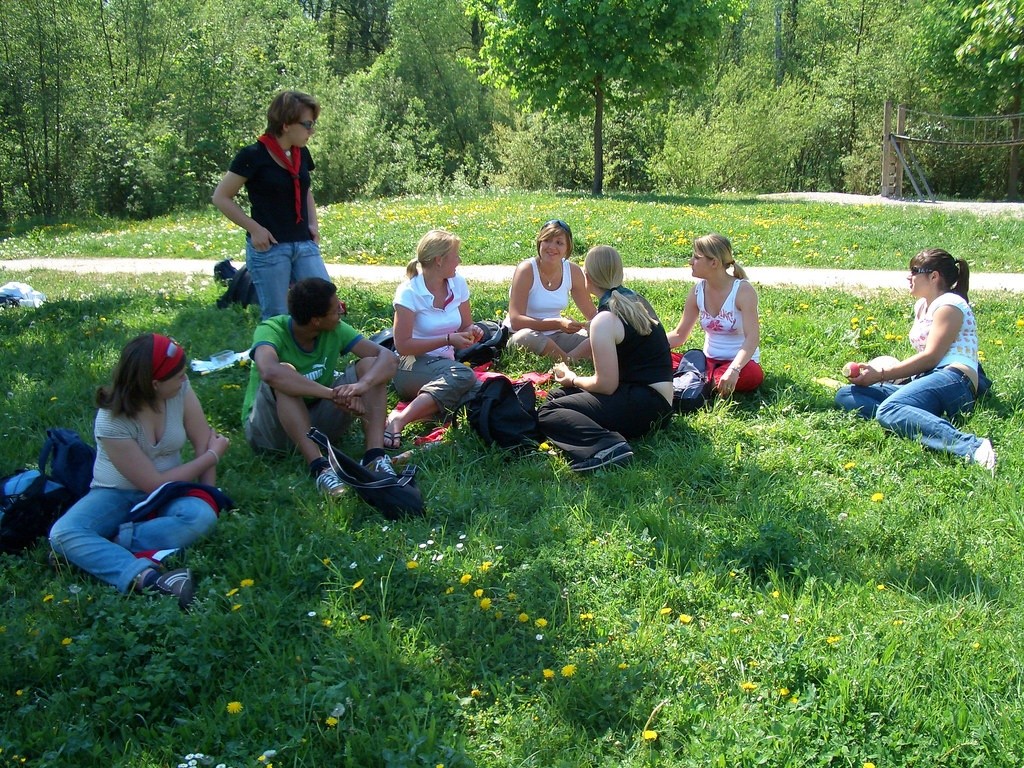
[384,431,401,450]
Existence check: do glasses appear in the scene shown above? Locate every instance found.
[296,120,314,129]
[153,335,177,379]
[542,220,570,232]
[912,267,941,276]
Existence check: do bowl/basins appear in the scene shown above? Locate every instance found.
[210,350,233,366]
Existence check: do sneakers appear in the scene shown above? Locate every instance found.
[155,567,192,610]
[570,441,634,474]
[361,452,395,476]
[315,468,349,500]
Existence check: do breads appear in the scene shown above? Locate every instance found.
[553,362,569,377]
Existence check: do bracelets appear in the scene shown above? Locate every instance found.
[880,368,884,388]
[208,449,219,464]
[447,334,449,343]
[728,366,740,372]
[572,378,576,386]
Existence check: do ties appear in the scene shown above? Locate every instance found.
[256,132,302,223]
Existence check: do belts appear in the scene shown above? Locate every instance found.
[944,366,977,398]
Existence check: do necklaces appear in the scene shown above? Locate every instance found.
[543,273,554,287]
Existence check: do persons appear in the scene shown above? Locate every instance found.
[666,234,763,397]
[836,248,995,469]
[537,245,674,471]
[50,334,230,608]
[503,220,599,364]
[213,91,331,319]
[241,277,396,497]
[386,229,484,450]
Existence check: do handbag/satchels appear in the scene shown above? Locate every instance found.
[309,425,425,521]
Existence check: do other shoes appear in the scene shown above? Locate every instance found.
[975,439,994,470]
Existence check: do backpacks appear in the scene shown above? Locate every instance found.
[217,264,258,309]
[0,469,68,550]
[215,260,237,287]
[39,428,97,499]
[454,320,509,364]
[451,376,541,456]
[672,349,709,411]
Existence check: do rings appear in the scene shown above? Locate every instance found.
[727,388,730,389]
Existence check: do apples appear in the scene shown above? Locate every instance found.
[841,362,860,378]
